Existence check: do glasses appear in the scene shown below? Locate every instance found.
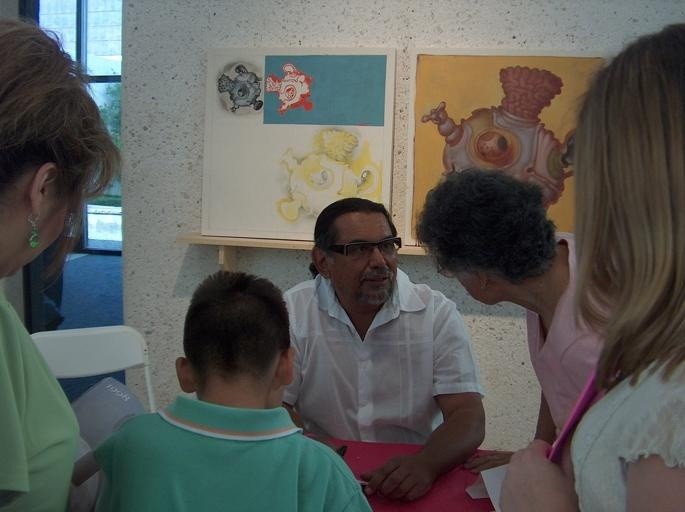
[321,236,402,260]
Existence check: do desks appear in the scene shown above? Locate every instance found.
[316,439,514,512]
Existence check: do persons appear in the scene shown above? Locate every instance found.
[273,197,497,511]
[1,10,118,511]
[64,269,377,512]
[415,166,613,442]
[499,23,684,511]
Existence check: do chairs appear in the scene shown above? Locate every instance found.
[29,325,155,412]
